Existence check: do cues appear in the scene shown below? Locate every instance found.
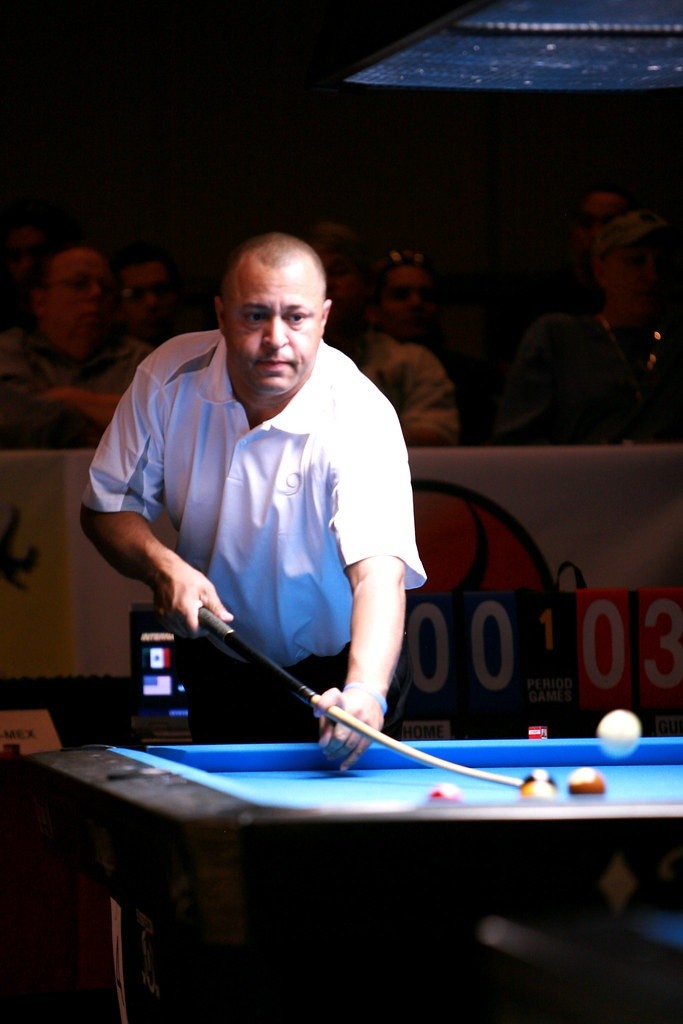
[198,607,525,788]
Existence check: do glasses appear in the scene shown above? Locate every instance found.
[120,282,178,300]
[45,275,118,298]
[383,283,438,303]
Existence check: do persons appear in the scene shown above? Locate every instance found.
[492,208,683,446]
[300,221,459,447]
[110,242,179,347]
[0,201,77,283]
[365,249,446,340]
[78,233,428,770]
[477,188,630,368]
[0,237,157,450]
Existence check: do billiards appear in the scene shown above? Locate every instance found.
[569,766,605,796]
[426,783,462,806]
[597,709,642,759]
[520,769,558,798]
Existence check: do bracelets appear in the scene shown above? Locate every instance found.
[343,683,387,715]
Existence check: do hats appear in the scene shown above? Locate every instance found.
[591,209,682,259]
